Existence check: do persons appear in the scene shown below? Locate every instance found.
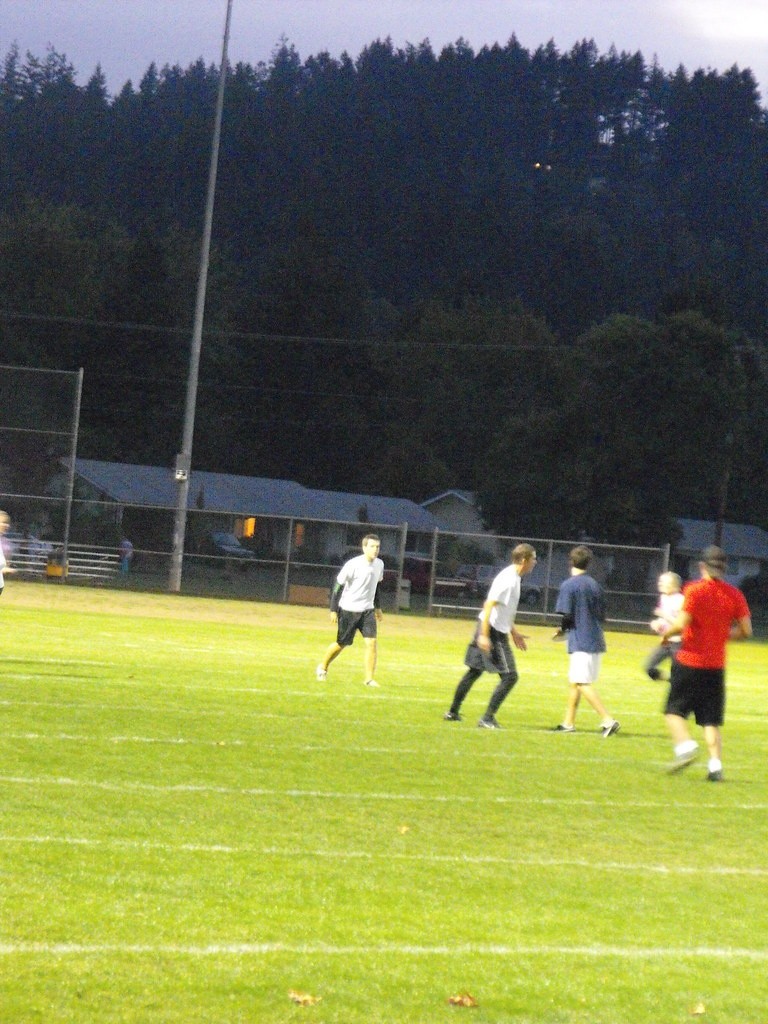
[317,533,384,686]
[644,544,753,780]
[551,545,620,736]
[119,537,134,579]
[0,510,13,595]
[443,543,537,729]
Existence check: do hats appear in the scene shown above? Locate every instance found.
[701,543,732,571]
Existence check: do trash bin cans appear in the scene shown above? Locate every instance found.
[398,579,412,610]
[378,568,401,613]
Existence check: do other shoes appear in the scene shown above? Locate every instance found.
[661,749,697,774]
[363,679,380,687]
[315,663,326,683]
[478,718,506,731]
[445,711,464,722]
[552,723,575,734]
[600,719,619,737]
[706,764,724,781]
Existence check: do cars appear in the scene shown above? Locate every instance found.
[186,529,256,570]
[338,548,542,607]
